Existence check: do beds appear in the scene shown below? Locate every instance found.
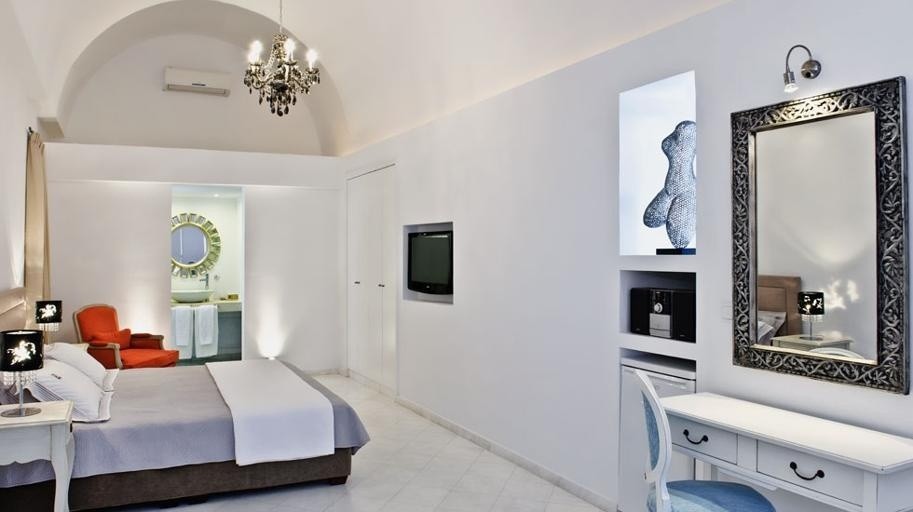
[753,274,804,347]
[0,340,372,510]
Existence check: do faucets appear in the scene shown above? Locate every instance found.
[199,273,210,289]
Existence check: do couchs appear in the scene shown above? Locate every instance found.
[73,303,179,369]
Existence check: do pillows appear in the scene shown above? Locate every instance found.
[755,321,773,342]
[757,308,787,345]
[13,338,119,421]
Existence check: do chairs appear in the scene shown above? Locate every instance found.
[636,366,774,510]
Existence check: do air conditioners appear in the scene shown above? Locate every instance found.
[159,64,234,98]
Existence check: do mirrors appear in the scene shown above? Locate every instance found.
[171,212,221,278]
[728,76,909,402]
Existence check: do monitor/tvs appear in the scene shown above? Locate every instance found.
[407,230,454,304]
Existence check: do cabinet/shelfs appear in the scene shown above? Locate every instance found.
[643,390,911,510]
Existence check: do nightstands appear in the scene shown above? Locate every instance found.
[0,400,78,510]
[769,331,855,356]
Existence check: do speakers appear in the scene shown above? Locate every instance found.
[630,288,652,336]
[649,288,695,339]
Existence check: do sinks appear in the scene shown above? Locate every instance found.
[171,289,212,303]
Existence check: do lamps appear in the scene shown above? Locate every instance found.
[782,44,824,95]
[1,330,47,416]
[244,1,324,118]
[796,290,826,342]
[34,299,63,343]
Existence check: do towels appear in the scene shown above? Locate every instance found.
[193,303,222,358]
[170,306,192,359]
